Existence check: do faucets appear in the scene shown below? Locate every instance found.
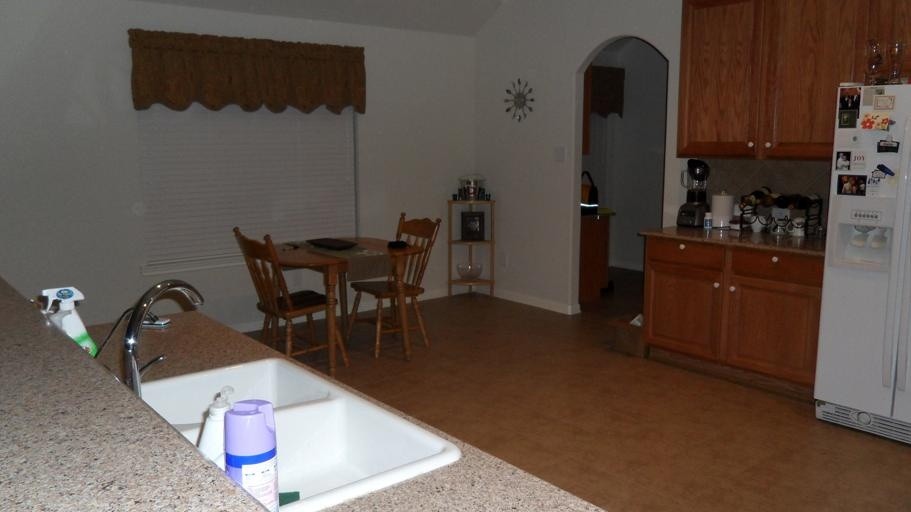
[120,278,207,400]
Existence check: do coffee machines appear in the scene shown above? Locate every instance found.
[676,160,710,229]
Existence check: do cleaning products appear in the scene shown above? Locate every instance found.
[39,285,98,357]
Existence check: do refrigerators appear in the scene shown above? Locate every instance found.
[809,80,911,445]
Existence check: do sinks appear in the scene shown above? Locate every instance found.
[178,397,462,512]
[140,355,335,428]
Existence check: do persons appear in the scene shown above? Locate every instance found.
[836,153,850,170]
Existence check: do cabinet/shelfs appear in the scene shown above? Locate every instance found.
[443,197,497,304]
[676,1,910,158]
[577,211,616,306]
[644,231,727,365]
[727,243,824,385]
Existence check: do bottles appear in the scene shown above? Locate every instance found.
[863,38,908,84]
[225,395,280,510]
[705,212,713,230]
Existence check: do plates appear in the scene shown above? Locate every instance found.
[307,235,358,252]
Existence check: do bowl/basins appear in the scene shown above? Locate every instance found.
[455,262,485,280]
[730,223,751,229]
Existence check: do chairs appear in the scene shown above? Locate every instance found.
[219,224,354,375]
[346,210,443,362]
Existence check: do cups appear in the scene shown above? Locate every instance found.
[751,217,767,230]
[451,187,492,201]
[786,217,805,237]
